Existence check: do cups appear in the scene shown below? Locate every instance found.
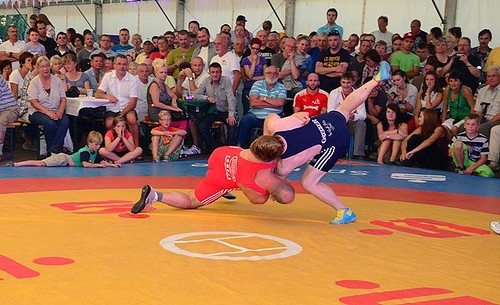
[86,88,93,100]
[189,89,195,100]
[182,90,188,100]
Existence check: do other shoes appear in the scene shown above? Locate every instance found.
[5,161,14,167]
[0,155,5,161]
[487,163,500,172]
[153,158,158,162]
[162,155,169,162]
[103,159,114,164]
[353,155,364,160]
[491,221,500,235]
[388,160,397,165]
[455,167,464,174]
[23,139,33,149]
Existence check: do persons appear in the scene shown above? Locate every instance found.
[317,8,343,39]
[132,146,295,214]
[0,14,500,171]
[150,110,187,162]
[6,131,121,168]
[249,60,391,224]
[450,115,495,178]
[99,116,142,164]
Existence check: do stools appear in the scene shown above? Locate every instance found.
[3,117,264,159]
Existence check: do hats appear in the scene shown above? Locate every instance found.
[403,32,417,40]
[327,30,340,36]
[237,15,247,22]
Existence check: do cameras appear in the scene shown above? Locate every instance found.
[454,53,463,57]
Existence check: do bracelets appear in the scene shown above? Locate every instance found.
[10,52,12,56]
[264,97,267,101]
[175,65,178,68]
[467,64,471,67]
[397,130,399,133]
[387,134,389,138]
[189,78,194,81]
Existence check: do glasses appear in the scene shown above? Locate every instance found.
[369,40,375,43]
[405,39,414,43]
[394,43,402,46]
[251,46,260,50]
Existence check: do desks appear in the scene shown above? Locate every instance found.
[65,96,110,151]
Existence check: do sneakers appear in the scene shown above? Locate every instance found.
[184,145,201,154]
[373,61,391,82]
[131,185,156,214]
[329,208,356,224]
[222,193,236,199]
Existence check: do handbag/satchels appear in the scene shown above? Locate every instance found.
[170,100,186,121]
